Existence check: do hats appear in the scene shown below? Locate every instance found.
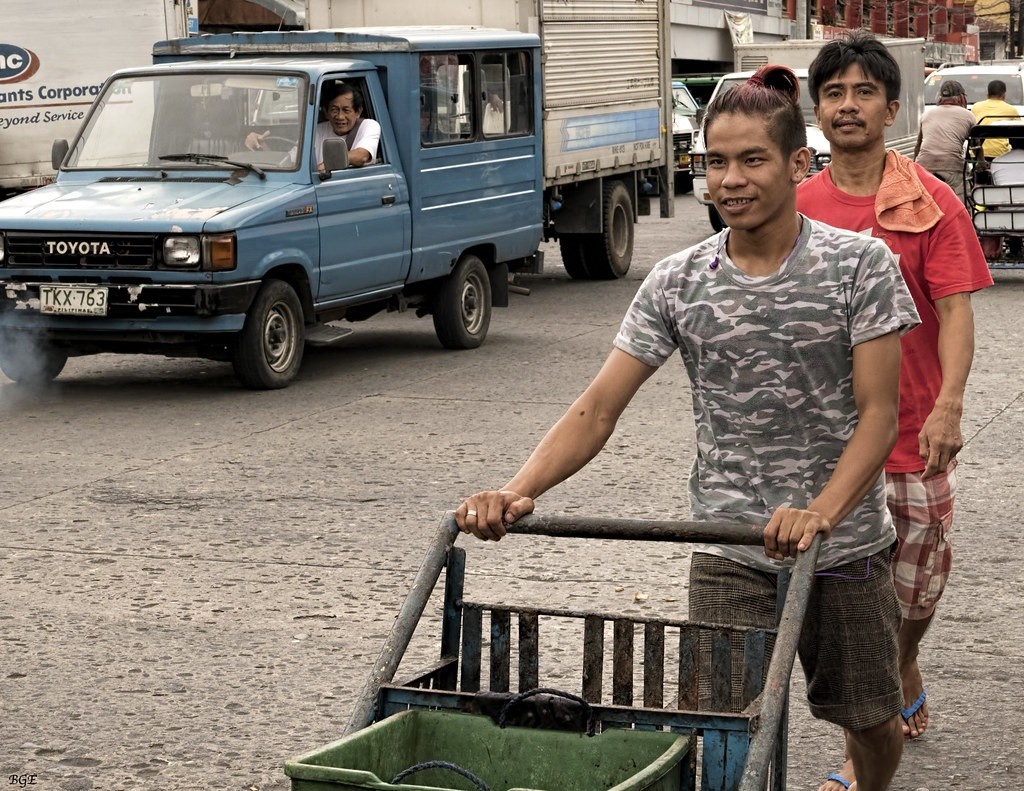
[941,80,967,97]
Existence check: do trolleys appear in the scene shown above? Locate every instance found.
[339,512,822,788]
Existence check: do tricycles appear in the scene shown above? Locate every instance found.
[933,116,1023,273]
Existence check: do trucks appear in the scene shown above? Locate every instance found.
[689,34,927,233]
[1,0,190,202]
[248,2,673,283]
[0,24,546,390]
[672,80,700,200]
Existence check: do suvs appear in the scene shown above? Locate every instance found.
[925,62,1024,118]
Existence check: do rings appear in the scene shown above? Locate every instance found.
[467,510,477,516]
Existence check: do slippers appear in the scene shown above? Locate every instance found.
[818,773,851,791]
[901,688,926,740]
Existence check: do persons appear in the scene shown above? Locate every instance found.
[794,36,996,791]
[967,79,1022,168]
[454,65,923,791]
[911,79,991,219]
[244,84,381,171]
